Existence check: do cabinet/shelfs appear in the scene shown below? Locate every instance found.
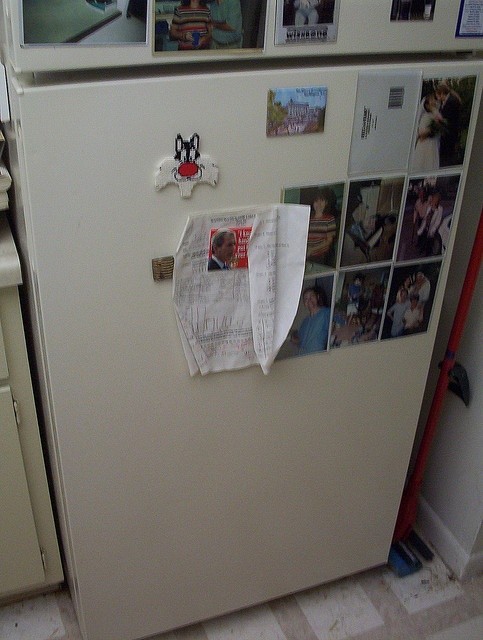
[0,287,66,605]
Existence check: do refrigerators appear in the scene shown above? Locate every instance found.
[1,2,483,639]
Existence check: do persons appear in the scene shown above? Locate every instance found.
[418,80,462,168]
[346,275,369,314]
[350,214,378,245]
[207,226,239,271]
[289,286,331,350]
[403,293,425,331]
[414,95,446,169]
[297,189,335,266]
[386,287,412,338]
[410,271,431,307]
[210,1,243,49]
[168,0,212,50]
[412,182,442,258]
